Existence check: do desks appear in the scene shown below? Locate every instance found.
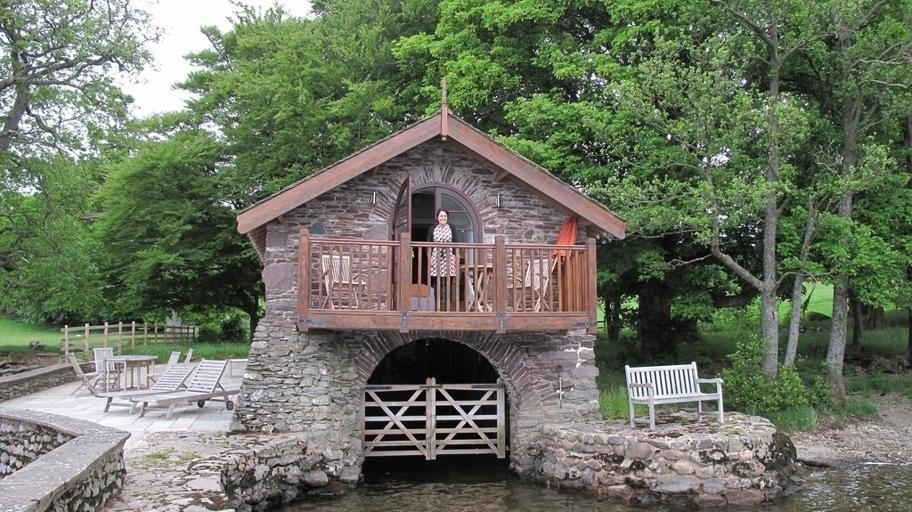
[461,266,494,312]
[106,354,159,392]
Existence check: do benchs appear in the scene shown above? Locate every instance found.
[624,361,725,428]
[228,358,249,377]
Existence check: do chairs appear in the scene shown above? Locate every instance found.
[97,363,196,415]
[322,255,367,310]
[129,356,241,420]
[67,353,104,396]
[145,348,194,389]
[507,258,554,312]
[93,348,123,393]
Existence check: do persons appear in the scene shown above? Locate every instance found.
[426,207,461,302]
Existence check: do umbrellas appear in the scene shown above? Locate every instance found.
[535,214,577,310]
